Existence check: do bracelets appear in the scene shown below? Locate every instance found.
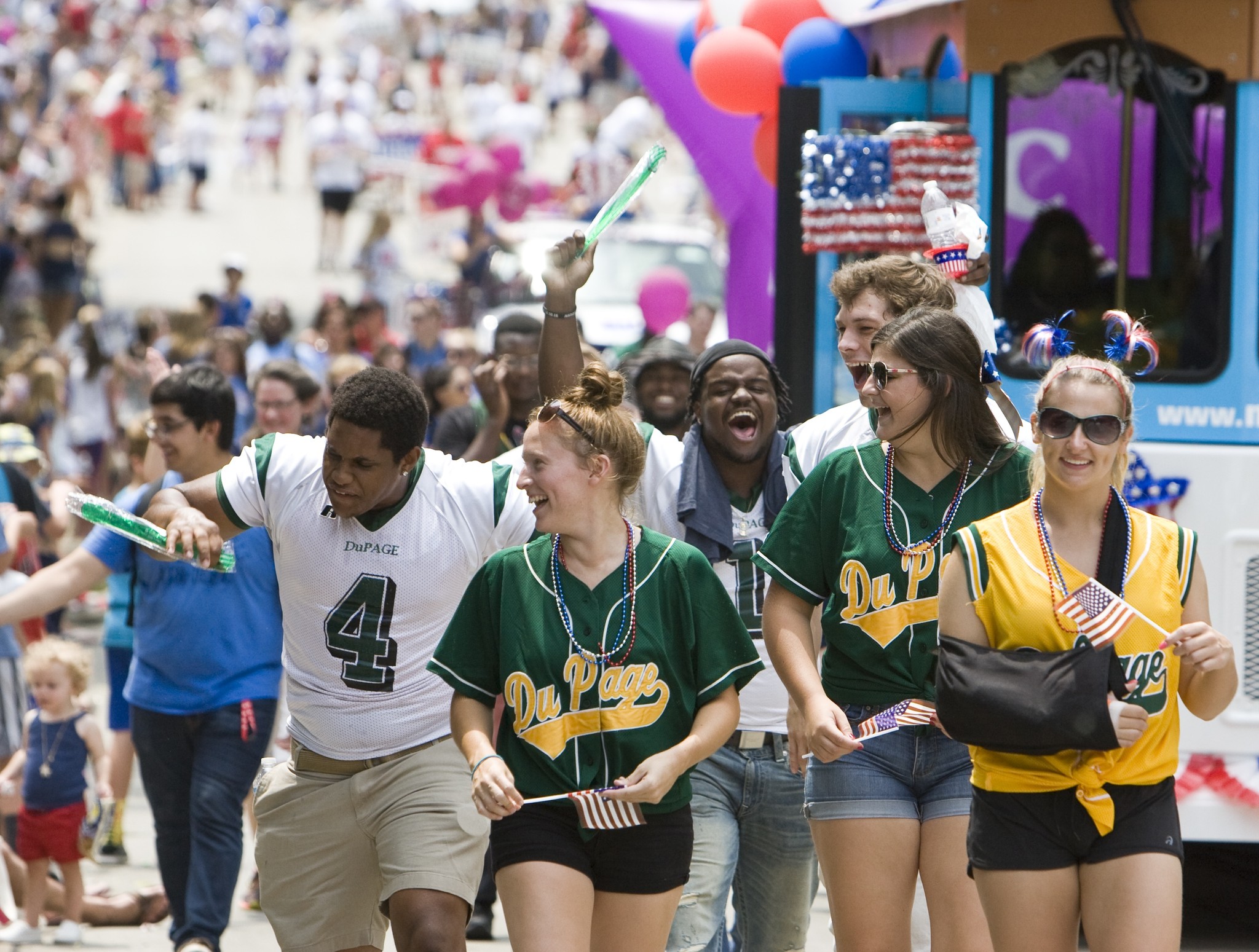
[543,304,577,318]
[471,754,504,781]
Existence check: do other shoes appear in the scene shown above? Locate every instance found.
[466,905,494,939]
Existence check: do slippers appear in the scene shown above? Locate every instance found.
[94,886,171,927]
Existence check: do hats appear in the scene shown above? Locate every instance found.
[0,423,49,470]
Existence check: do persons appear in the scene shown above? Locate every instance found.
[0,0,712,926]
[939,354,1237,952]
[142,367,549,952]
[759,309,1038,952]
[425,364,766,952]
[1007,207,1106,348]
[540,230,1023,952]
[782,257,1015,677]
[0,361,282,952]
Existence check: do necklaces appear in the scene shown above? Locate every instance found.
[1034,488,1131,633]
[550,517,637,666]
[883,448,969,555]
[33,711,70,778]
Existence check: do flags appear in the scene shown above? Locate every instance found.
[570,578,1134,831]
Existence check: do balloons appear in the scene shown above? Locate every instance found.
[429,0,967,346]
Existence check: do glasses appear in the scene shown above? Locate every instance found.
[1037,407,1128,445]
[252,397,298,412]
[143,418,193,442]
[537,398,603,454]
[864,361,919,390]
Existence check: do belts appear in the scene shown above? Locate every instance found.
[724,730,789,751]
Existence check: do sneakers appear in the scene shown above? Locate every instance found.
[88,798,128,865]
[54,920,83,944]
[0,920,43,944]
